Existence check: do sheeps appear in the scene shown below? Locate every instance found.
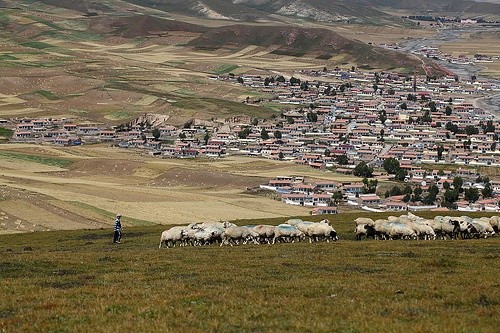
[353,211,500,241]
[158,219,339,249]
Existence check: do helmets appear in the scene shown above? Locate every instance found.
[116,213,122,217]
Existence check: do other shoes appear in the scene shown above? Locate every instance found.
[116,241,121,243]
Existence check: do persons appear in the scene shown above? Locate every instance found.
[113,214,124,244]
